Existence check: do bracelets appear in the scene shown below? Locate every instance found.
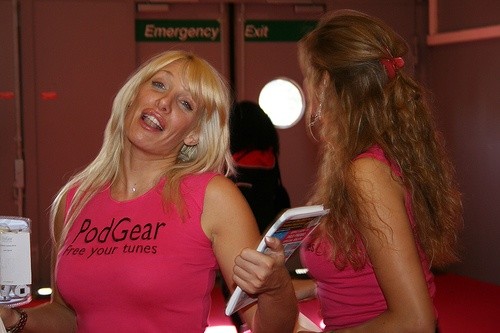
[5,308,28,333]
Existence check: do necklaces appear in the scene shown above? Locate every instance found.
[115,171,162,192]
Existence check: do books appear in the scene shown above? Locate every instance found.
[226,203,330,318]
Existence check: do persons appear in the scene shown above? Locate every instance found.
[292,8,463,333]
[0,47,300,333]
